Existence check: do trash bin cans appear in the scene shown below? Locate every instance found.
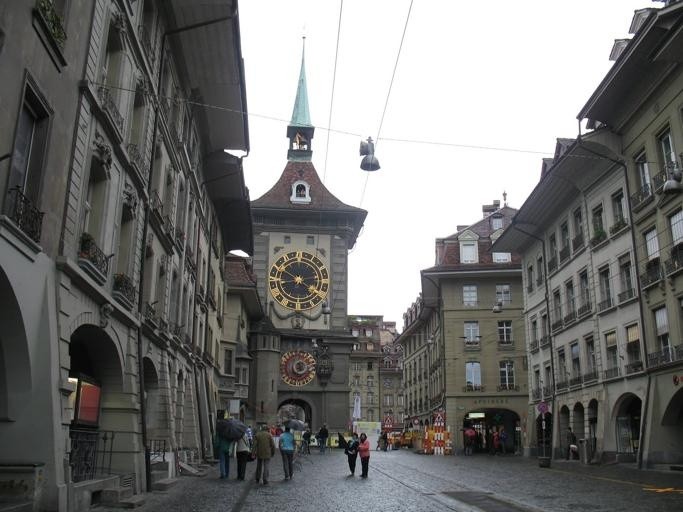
[578,438,592,464]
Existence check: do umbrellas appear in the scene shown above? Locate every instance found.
[282,417,307,431]
[337,431,349,450]
[463,428,478,437]
[215,417,247,443]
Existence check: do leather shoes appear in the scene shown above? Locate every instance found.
[263,480,268,485]
[255,479,259,484]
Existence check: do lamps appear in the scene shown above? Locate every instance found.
[360,137,381,171]
[312,337,318,348]
[321,301,331,315]
[492,301,502,312]
[663,164,683,194]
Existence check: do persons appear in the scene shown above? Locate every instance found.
[214,434,234,479]
[565,426,578,460]
[319,423,328,455]
[314,425,323,449]
[383,430,388,451]
[278,426,297,479]
[343,431,359,477]
[302,427,310,454]
[356,433,369,480]
[375,431,384,451]
[492,430,503,456]
[245,425,253,451]
[231,432,255,481]
[270,424,275,435]
[486,428,495,456]
[251,424,276,483]
[274,424,283,436]
[500,428,509,454]
[464,435,475,456]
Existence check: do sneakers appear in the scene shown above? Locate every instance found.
[284,476,290,480]
[290,473,293,479]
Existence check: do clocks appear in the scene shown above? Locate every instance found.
[268,250,329,311]
